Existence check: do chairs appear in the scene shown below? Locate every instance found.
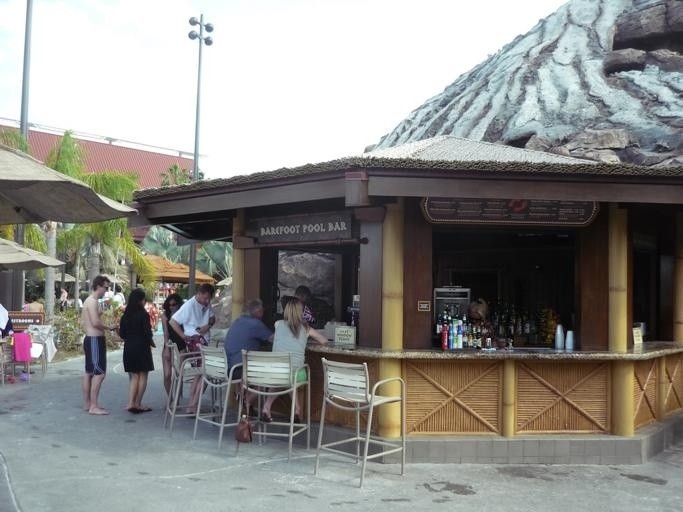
[164,339,201,429]
[236,348,312,455]
[25,325,54,374]
[318,358,407,488]
[190,344,250,445]
[2,335,29,385]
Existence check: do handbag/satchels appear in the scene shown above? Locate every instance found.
[235,414,253,442]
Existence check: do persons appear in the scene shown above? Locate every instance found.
[161,294,187,410]
[296,286,317,324]
[262,297,328,423]
[116,289,156,412]
[224,298,274,415]
[81,277,115,415]
[169,284,215,414]
[22,295,43,312]
[113,286,125,305]
[60,286,68,311]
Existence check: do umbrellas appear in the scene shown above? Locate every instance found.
[85,274,125,283]
[216,277,232,286]
[0,144,139,226]
[41,273,81,282]
[0,238,66,270]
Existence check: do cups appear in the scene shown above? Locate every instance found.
[554,323,573,349]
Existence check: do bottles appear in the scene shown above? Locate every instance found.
[435,309,491,350]
[492,302,530,337]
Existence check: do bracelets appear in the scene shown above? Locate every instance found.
[208,322,212,327]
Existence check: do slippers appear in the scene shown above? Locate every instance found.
[260,413,273,422]
[128,406,152,413]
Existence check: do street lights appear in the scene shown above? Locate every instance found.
[186,11,216,301]
